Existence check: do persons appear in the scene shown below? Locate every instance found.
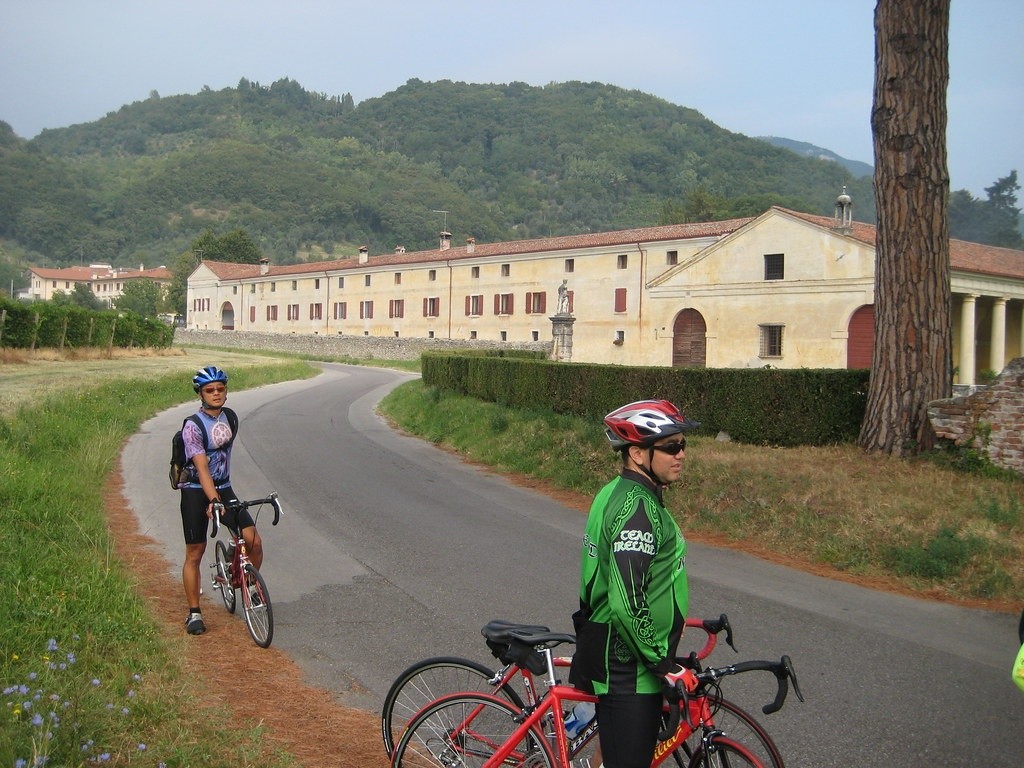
[176,366,264,635]
[1012,608,1024,693]
[557,279,569,314]
[567,399,700,768]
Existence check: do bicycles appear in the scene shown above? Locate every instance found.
[209,491,284,649]
[382,614,805,768]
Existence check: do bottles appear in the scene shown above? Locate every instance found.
[227,542,236,562]
[563,701,596,740]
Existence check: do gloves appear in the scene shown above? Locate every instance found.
[663,663,698,693]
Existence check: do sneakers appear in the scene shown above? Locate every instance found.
[185,613,206,635]
[248,585,261,606]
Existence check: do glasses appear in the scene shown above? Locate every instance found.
[650,440,686,455]
[202,385,225,393]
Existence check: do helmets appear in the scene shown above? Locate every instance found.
[603,400,693,452]
[192,367,228,393]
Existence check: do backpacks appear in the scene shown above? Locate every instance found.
[168,407,239,490]
